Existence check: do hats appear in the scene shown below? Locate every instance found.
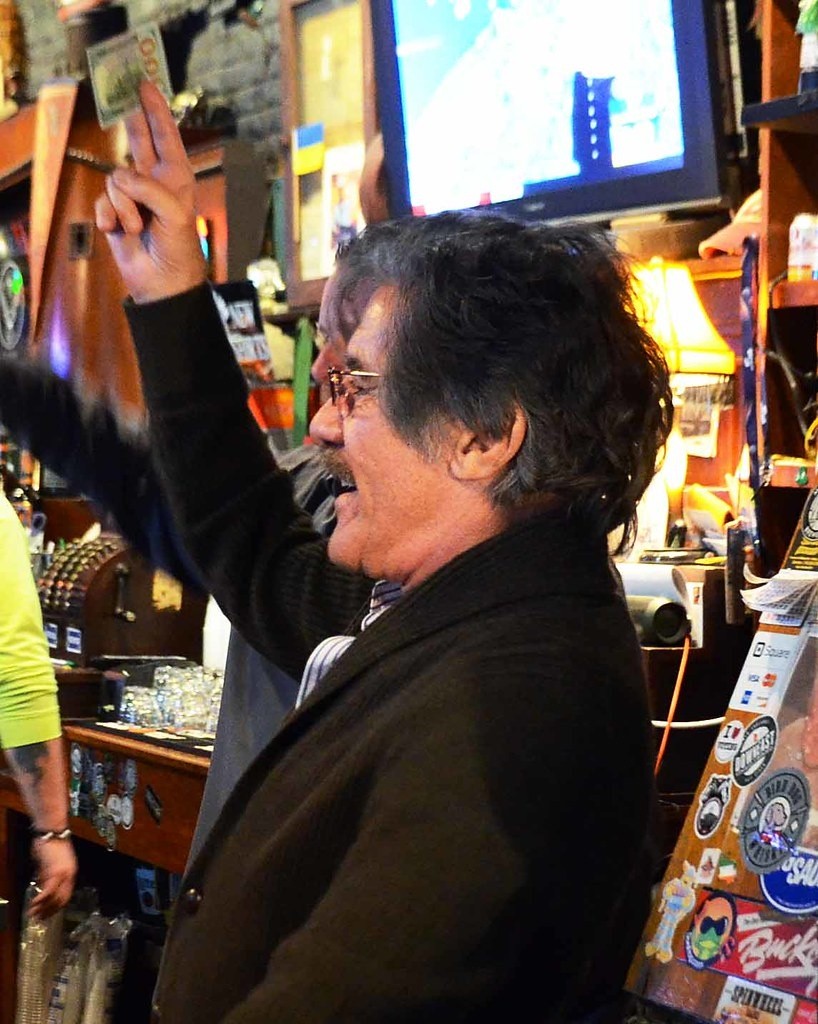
[698,188,762,260]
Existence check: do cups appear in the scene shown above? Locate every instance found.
[119,665,226,734]
[15,903,128,1023]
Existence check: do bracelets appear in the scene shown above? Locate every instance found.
[27,821,75,845]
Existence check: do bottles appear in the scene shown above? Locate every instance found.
[785,213,818,282]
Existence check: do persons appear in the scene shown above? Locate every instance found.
[4,215,486,912]
[0,469,81,1024]
[94,80,680,1023]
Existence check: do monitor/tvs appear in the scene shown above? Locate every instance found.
[368,1,730,224]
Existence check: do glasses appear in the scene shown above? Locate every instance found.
[326,365,384,407]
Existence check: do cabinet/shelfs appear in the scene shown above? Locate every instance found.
[0,498,210,1024]
